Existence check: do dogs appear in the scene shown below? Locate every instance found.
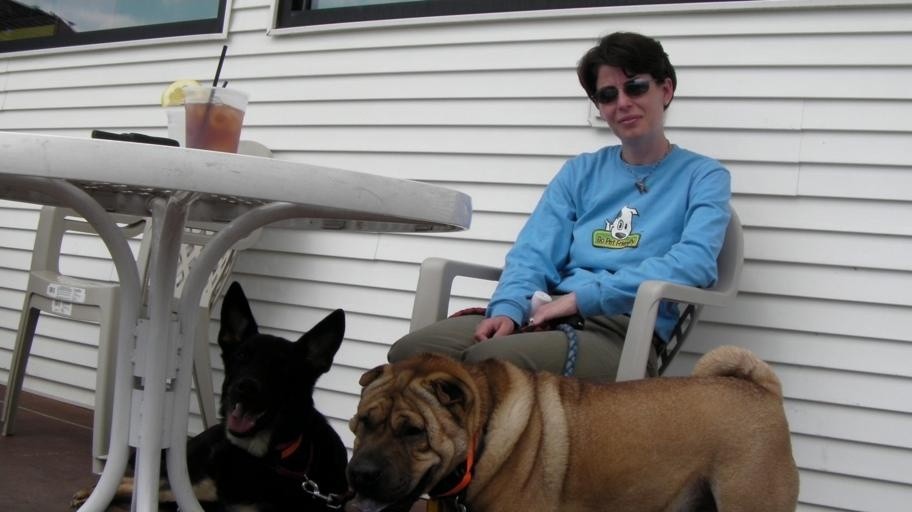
[69,279,349,511]
[344,344,801,511]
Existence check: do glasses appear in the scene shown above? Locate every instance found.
[590,75,663,104]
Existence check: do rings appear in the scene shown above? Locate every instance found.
[527,317,535,325]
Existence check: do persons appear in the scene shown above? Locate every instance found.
[382,29,735,387]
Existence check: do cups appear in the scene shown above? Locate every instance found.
[166,106,186,147]
[183,86,249,154]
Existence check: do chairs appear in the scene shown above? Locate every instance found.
[0,126,471,512]
[408,202,745,384]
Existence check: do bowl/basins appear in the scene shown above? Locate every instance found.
[237,139,273,159]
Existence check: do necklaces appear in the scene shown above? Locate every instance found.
[615,137,674,197]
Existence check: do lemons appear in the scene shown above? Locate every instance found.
[162,78,202,108]
